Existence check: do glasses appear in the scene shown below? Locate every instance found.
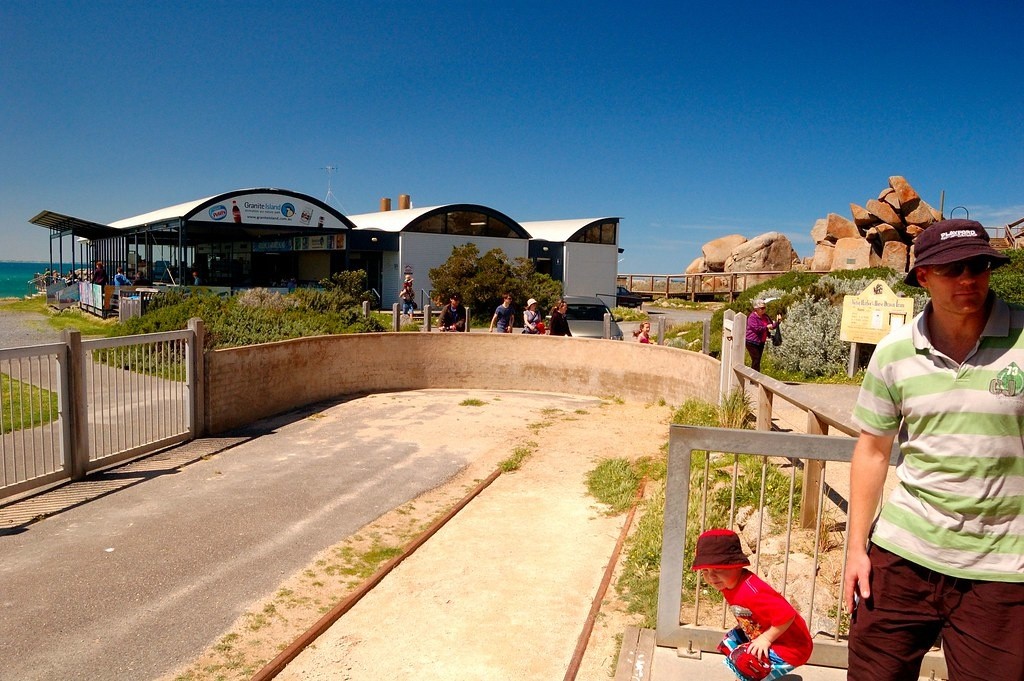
[926,258,991,278]
[508,298,512,300]
[760,307,766,310]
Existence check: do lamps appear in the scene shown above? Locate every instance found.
[618,247,624,253]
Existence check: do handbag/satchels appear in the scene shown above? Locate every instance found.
[412,302,417,309]
[522,329,528,334]
[773,321,781,345]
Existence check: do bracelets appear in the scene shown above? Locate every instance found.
[759,632,772,644]
[508,325,512,328]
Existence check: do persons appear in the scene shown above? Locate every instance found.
[549,300,572,337]
[523,299,541,334]
[43,263,298,305]
[633,322,653,344]
[746,299,781,387]
[842,218,1023,681]
[436,292,466,332]
[398,274,415,321]
[691,529,814,681]
[489,292,514,333]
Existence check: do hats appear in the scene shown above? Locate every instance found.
[692,529,751,570]
[753,300,766,308]
[193,271,198,275]
[451,293,460,300]
[903,219,1011,287]
[527,299,537,307]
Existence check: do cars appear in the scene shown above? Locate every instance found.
[616,286,643,311]
[544,296,623,340]
